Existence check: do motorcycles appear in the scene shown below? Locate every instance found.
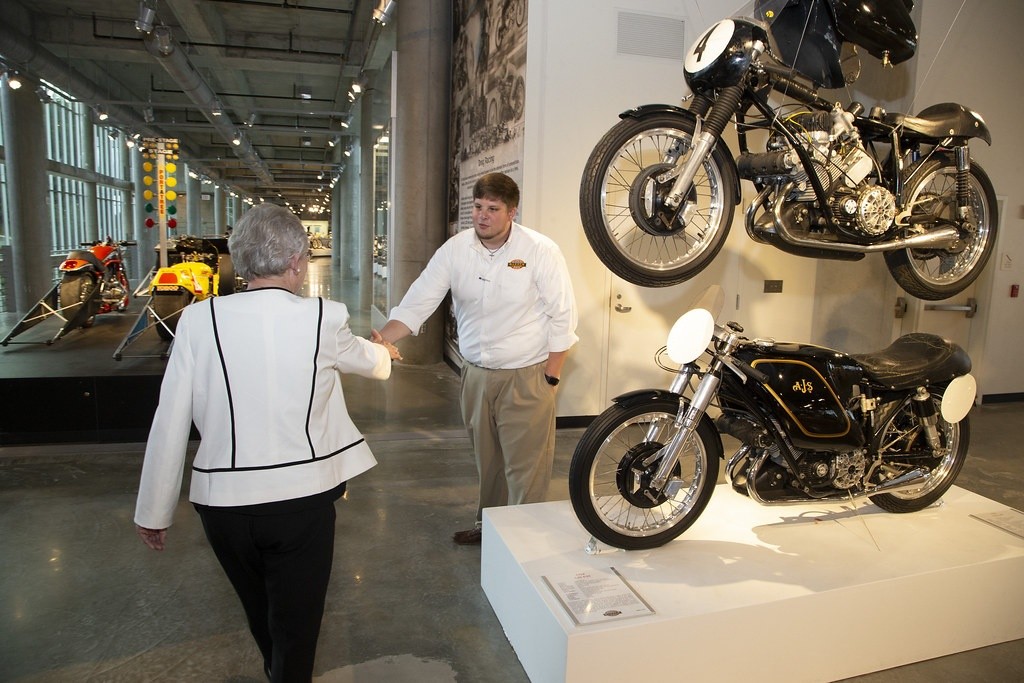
[578,0,999,301]
[59,235,137,328]
[569,284,977,551]
[149,236,220,341]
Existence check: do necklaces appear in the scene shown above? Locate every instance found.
[480,237,508,260]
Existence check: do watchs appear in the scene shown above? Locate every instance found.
[545,373,560,386]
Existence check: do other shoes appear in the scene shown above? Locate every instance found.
[451,521,482,546]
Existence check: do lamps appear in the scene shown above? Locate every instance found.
[96,110,108,121]
[317,77,364,188]
[211,99,222,115]
[152,26,174,55]
[6,70,22,89]
[125,138,135,147]
[34,87,51,104]
[134,6,156,35]
[372,0,397,27]
[108,130,119,141]
[142,107,155,123]
[233,132,242,145]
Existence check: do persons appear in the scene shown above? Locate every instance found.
[370,172,579,544]
[134,202,403,683]
